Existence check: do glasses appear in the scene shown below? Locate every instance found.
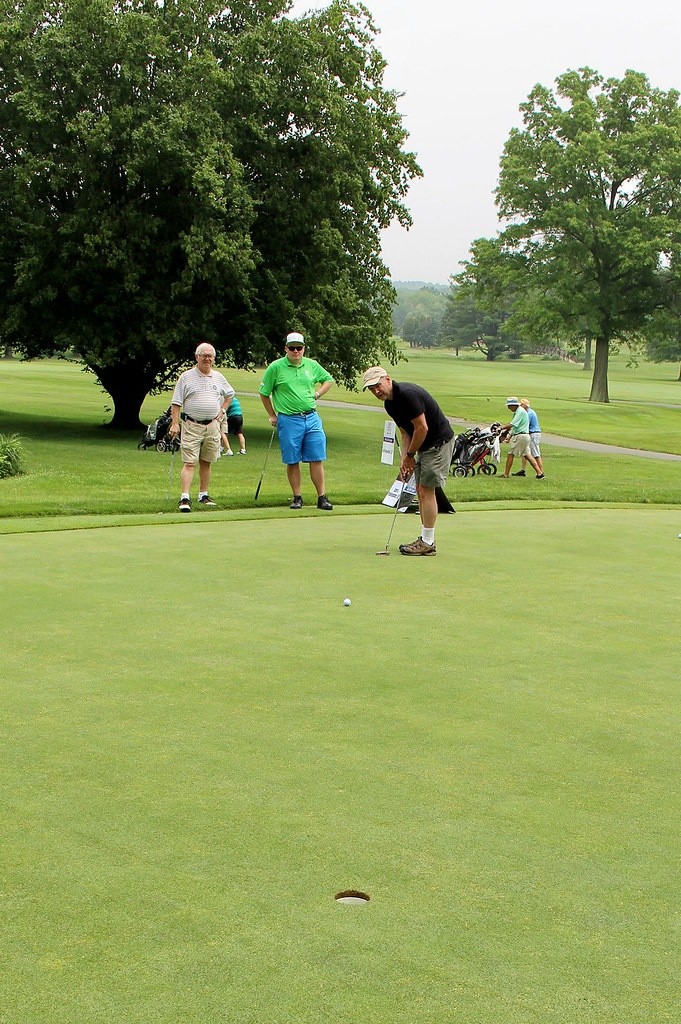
[285,345,304,351]
[196,352,215,359]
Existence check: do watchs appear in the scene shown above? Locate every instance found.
[406,451,416,458]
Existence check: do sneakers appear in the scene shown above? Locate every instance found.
[399,538,437,555]
[399,535,424,551]
[316,494,334,510]
[199,495,217,507]
[290,495,303,509]
[178,498,191,511]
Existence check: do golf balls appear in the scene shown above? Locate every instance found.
[344,598,351,606]
[679,534,681,539]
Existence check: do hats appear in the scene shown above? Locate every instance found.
[286,332,305,346]
[503,396,520,407]
[361,366,387,392]
[520,399,530,407]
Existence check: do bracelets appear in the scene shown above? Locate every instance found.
[316,392,320,396]
[222,409,226,414]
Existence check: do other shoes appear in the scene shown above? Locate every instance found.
[223,451,233,455]
[534,473,545,480]
[237,450,246,455]
[494,474,508,479]
[511,471,526,476]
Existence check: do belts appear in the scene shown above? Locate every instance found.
[181,412,216,425]
[513,432,526,436]
[295,409,316,415]
[529,430,540,434]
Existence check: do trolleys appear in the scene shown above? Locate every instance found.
[138,405,181,453]
[450,421,511,478]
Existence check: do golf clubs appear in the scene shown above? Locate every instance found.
[163,410,172,423]
[463,427,481,443]
[376,469,409,556]
[255,419,276,501]
[158,437,177,515]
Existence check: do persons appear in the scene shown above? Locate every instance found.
[361,367,455,555]
[497,397,545,480]
[220,412,233,456]
[258,332,336,510]
[220,397,247,455]
[511,399,544,476]
[170,343,238,512]
